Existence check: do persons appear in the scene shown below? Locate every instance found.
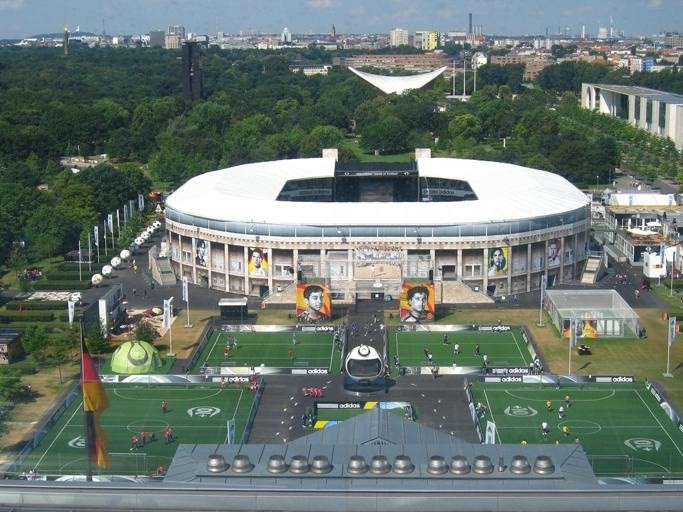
[488,249,506,276]
[350,313,384,346]
[133,264,137,275]
[453,342,460,354]
[300,384,324,398]
[287,348,293,359]
[427,352,432,365]
[297,286,329,322]
[574,436,580,444]
[546,399,551,410]
[424,345,428,359]
[132,287,135,296]
[247,375,257,392]
[16,469,41,482]
[393,354,399,368]
[400,286,433,322]
[353,245,400,279]
[160,399,167,413]
[220,376,225,388]
[132,257,136,267]
[442,333,448,344]
[558,406,563,419]
[565,393,570,407]
[247,249,266,277]
[547,240,560,267]
[195,240,206,267]
[236,378,243,388]
[292,332,296,346]
[143,288,147,299]
[520,439,527,445]
[562,424,568,437]
[223,335,239,360]
[155,464,164,475]
[301,412,307,427]
[474,344,479,355]
[482,353,488,366]
[614,272,652,301]
[128,425,172,451]
[554,376,560,391]
[540,421,548,436]
[151,280,154,289]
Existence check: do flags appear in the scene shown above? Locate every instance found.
[80,332,107,468]
